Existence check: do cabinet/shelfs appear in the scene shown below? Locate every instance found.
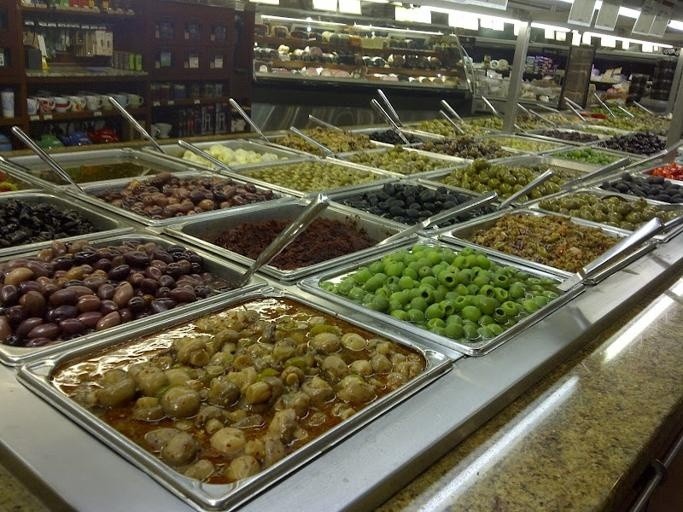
[0,0,250,151]
[251,6,471,136]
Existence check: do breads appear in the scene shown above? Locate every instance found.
[252,24,458,85]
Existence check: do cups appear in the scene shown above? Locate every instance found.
[27,89,144,114]
[150,122,171,140]
